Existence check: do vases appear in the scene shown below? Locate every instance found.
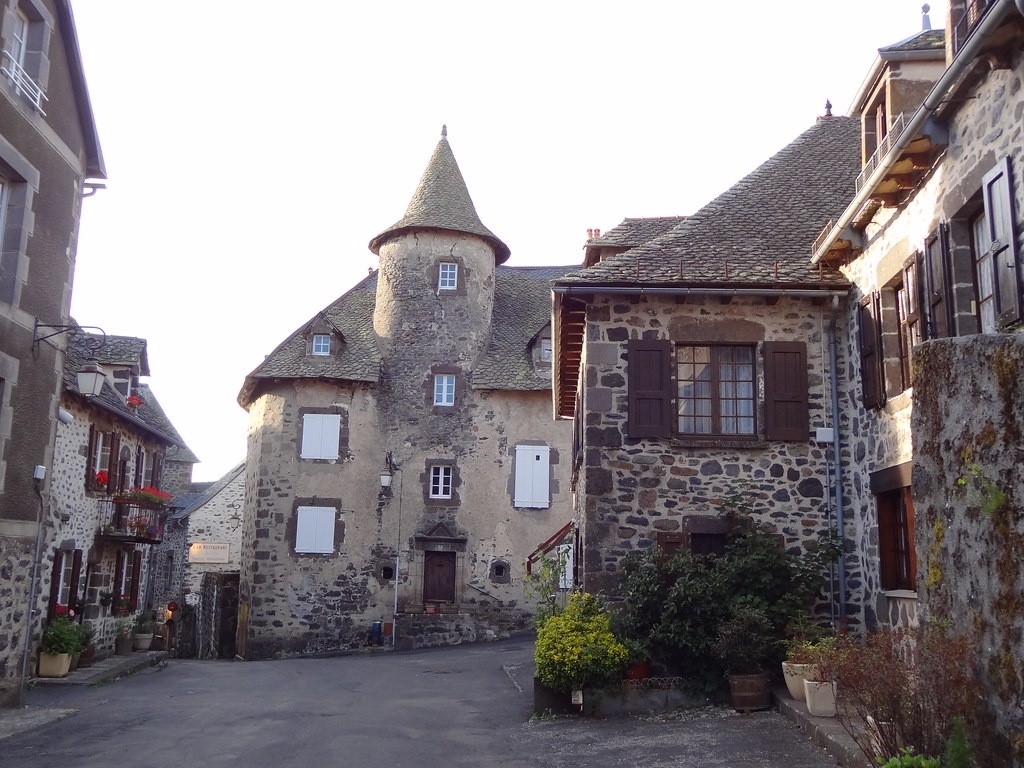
[39,652,72,677]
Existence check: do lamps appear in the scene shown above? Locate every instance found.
[380,452,392,489]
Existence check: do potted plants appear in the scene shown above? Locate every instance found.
[804,637,845,717]
[623,638,651,686]
[782,610,824,699]
[113,620,135,653]
[713,609,778,710]
[134,613,155,649]
[82,624,95,657]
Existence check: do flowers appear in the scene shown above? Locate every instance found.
[123,515,165,538]
[131,486,170,505]
[96,470,109,485]
[43,609,78,654]
